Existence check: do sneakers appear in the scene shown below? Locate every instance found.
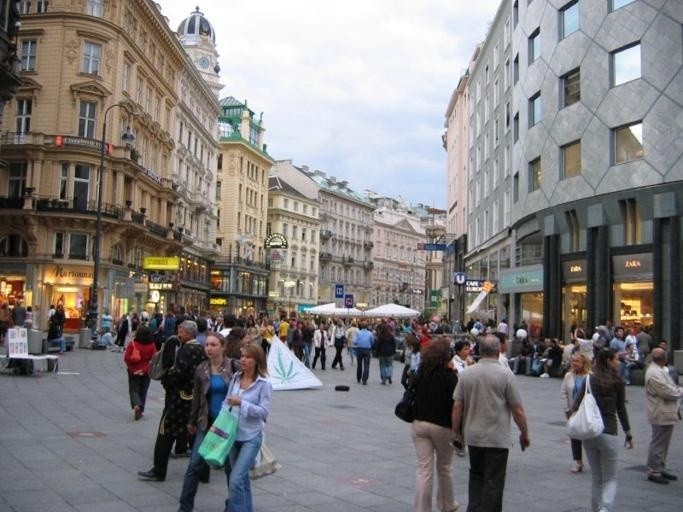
[540,372,548,377]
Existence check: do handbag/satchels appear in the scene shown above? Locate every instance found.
[198,396,239,467]
[566,374,604,440]
[395,371,420,422]
[128,340,141,363]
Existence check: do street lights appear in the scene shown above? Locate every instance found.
[88,105,135,329]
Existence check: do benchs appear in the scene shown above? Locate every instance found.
[511,340,645,386]
[4,326,60,378]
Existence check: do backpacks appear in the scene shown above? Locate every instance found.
[147,336,182,380]
[248,440,281,479]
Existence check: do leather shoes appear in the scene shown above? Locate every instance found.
[648,475,669,483]
[661,471,677,480]
[135,406,141,420]
[138,450,209,483]
[438,501,459,511]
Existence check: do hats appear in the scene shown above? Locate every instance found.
[595,326,607,330]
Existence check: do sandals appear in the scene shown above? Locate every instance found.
[571,463,583,472]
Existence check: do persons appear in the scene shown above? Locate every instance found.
[444,340,477,457]
[451,334,530,512]
[561,352,593,471]
[218,342,273,512]
[574,348,633,512]
[178,332,233,511]
[162,315,196,457]
[97,301,676,422]
[139,320,209,482]
[0,299,32,342]
[411,339,460,512]
[48,305,67,355]
[646,348,683,484]
